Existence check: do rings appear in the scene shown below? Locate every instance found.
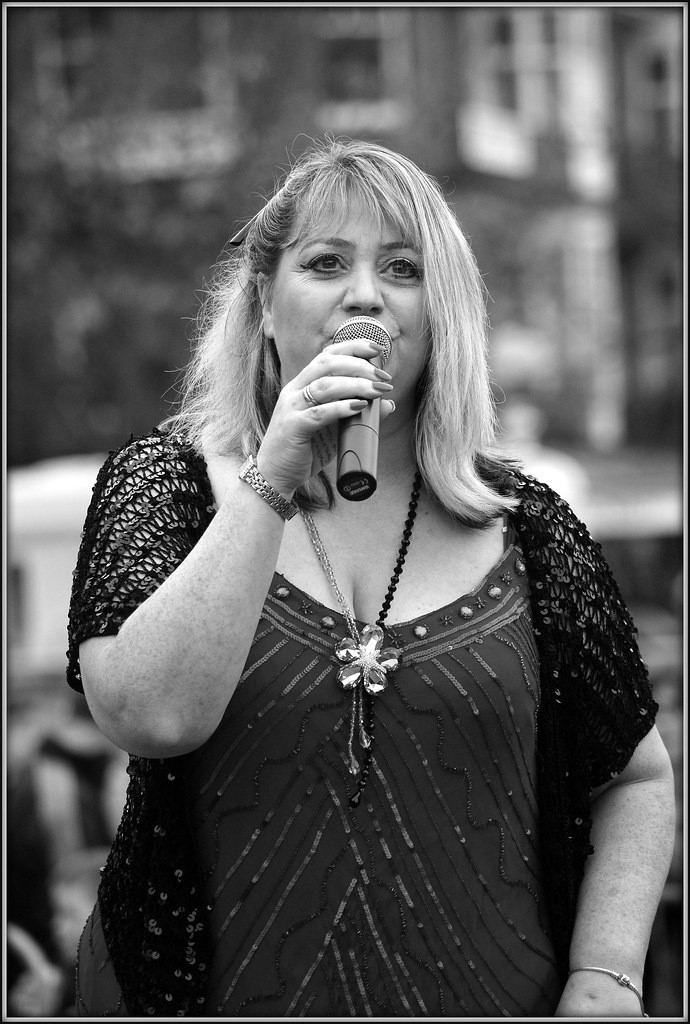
[302,384,318,407]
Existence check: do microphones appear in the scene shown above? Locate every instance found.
[332,315,393,503]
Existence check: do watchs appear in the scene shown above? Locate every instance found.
[238,455,300,521]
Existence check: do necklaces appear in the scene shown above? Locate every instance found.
[300,463,423,808]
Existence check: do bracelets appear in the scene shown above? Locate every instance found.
[567,967,644,1017]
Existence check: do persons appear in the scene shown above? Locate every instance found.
[67,140,675,1018]
